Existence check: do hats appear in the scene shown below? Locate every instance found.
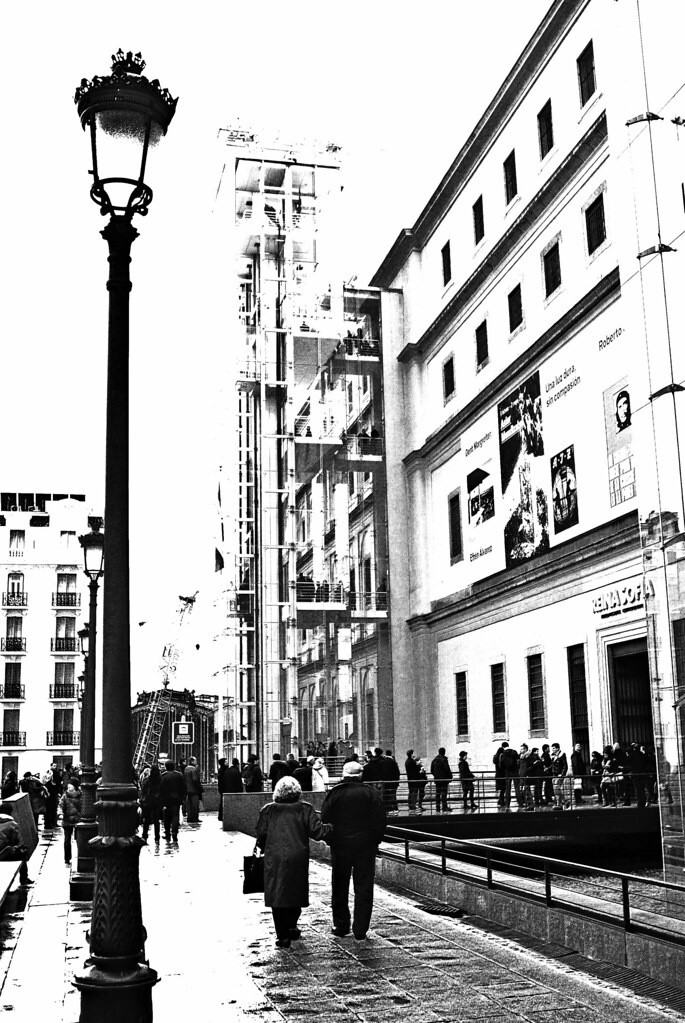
[67,778,79,789]
[307,755,314,762]
[459,751,467,758]
[24,771,31,778]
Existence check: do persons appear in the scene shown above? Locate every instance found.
[375,578,386,610]
[339,426,379,455]
[570,743,587,804]
[431,747,454,811]
[300,321,309,332]
[238,578,249,612]
[255,776,333,948]
[590,742,674,807]
[458,751,479,808]
[295,425,312,437]
[321,761,388,944]
[616,391,632,433]
[493,742,571,810]
[0,755,205,885]
[343,328,380,356]
[295,573,344,603]
[218,736,428,821]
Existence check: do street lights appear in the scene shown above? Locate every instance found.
[74,47,176,1021]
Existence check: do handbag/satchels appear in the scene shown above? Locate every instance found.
[573,778,582,790]
[603,771,625,784]
[242,841,269,893]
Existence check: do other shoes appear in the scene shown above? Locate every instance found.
[162,836,171,842]
[331,926,350,936]
[172,841,178,846]
[289,933,297,941]
[183,814,202,822]
[275,940,291,948]
[37,814,61,831]
[22,879,35,885]
[64,861,72,866]
[353,937,367,944]
